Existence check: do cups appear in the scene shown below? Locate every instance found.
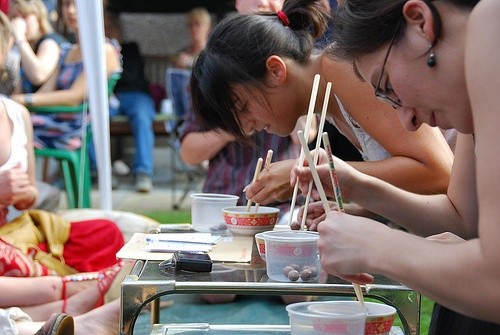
[285,300,370,335]
[190,193,240,234]
[262,230,321,283]
[307,301,397,335]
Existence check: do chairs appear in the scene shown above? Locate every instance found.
[27,68,209,214]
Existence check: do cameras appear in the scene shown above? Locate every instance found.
[172,250,212,272]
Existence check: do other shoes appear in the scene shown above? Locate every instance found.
[35,312,74,335]
[111,176,118,188]
[135,174,152,191]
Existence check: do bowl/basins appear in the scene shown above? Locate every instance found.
[255,231,320,265]
[221,205,280,237]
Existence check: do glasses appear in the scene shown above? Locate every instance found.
[374,17,410,109]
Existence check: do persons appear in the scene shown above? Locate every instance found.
[1,0,156,335]
[188,1,459,206]
[297,0,500,335]
[174,1,366,208]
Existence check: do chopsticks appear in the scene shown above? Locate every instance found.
[296,130,366,310]
[289,71,332,230]
[246,149,273,212]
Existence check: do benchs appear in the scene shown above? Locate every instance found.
[109,56,174,135]
